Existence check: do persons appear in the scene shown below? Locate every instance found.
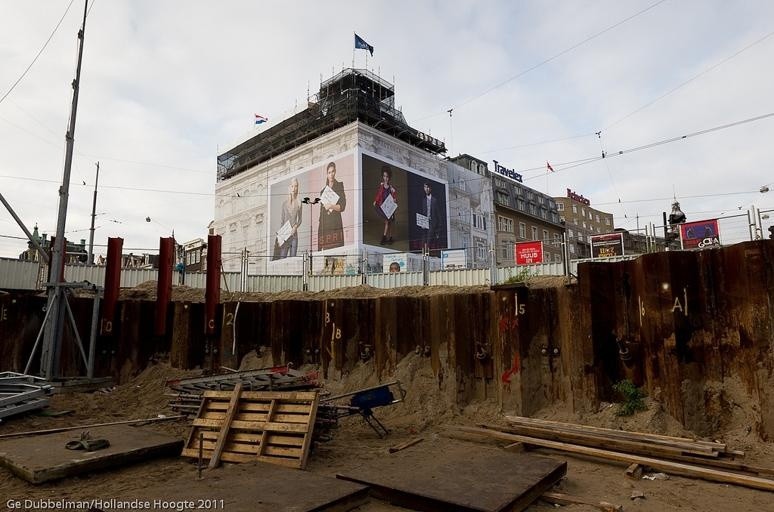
[388,262,400,273]
[417,181,439,248]
[687,227,694,239]
[318,163,346,249]
[701,225,713,237]
[369,167,398,244]
[274,177,303,258]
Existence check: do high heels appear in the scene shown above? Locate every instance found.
[380,236,394,245]
